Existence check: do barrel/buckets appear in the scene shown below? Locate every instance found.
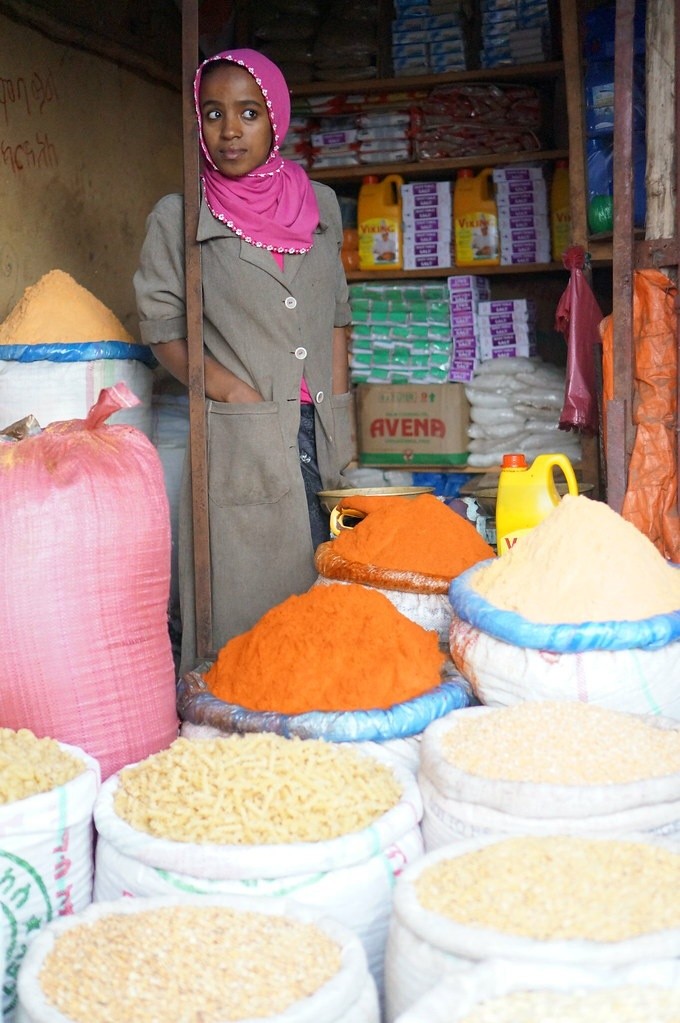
[356,174,403,271]
[495,453,579,556]
[453,168,501,267]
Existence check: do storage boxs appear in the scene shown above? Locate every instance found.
[399,181,455,271]
[448,275,539,382]
[493,162,554,266]
[392,0,561,78]
[356,382,471,469]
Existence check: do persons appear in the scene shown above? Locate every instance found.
[132,49,357,681]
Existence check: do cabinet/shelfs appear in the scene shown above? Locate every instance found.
[236,0,602,505]
